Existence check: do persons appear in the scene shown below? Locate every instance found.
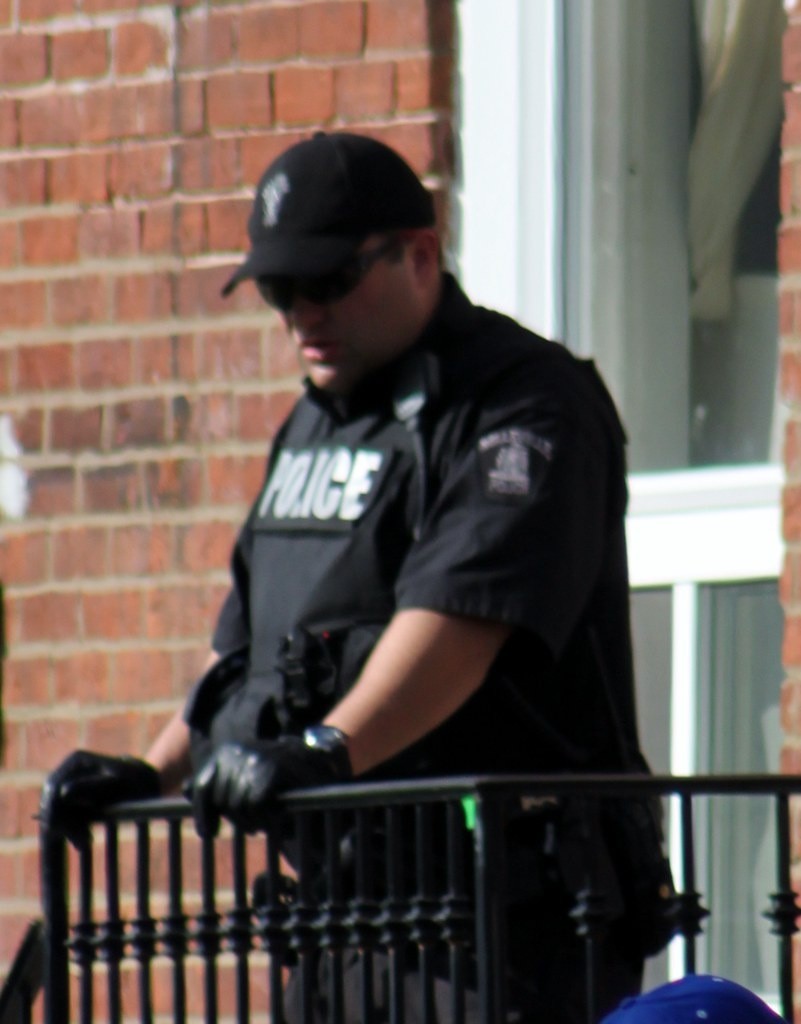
[37,133,676,1024]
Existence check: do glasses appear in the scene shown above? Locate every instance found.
[245,244,395,303]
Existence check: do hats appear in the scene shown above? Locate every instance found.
[218,130,434,303]
[600,973,790,1024]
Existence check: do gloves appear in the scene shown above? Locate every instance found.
[178,735,337,840]
[38,746,163,850]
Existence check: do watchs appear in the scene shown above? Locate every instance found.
[304,724,354,781]
